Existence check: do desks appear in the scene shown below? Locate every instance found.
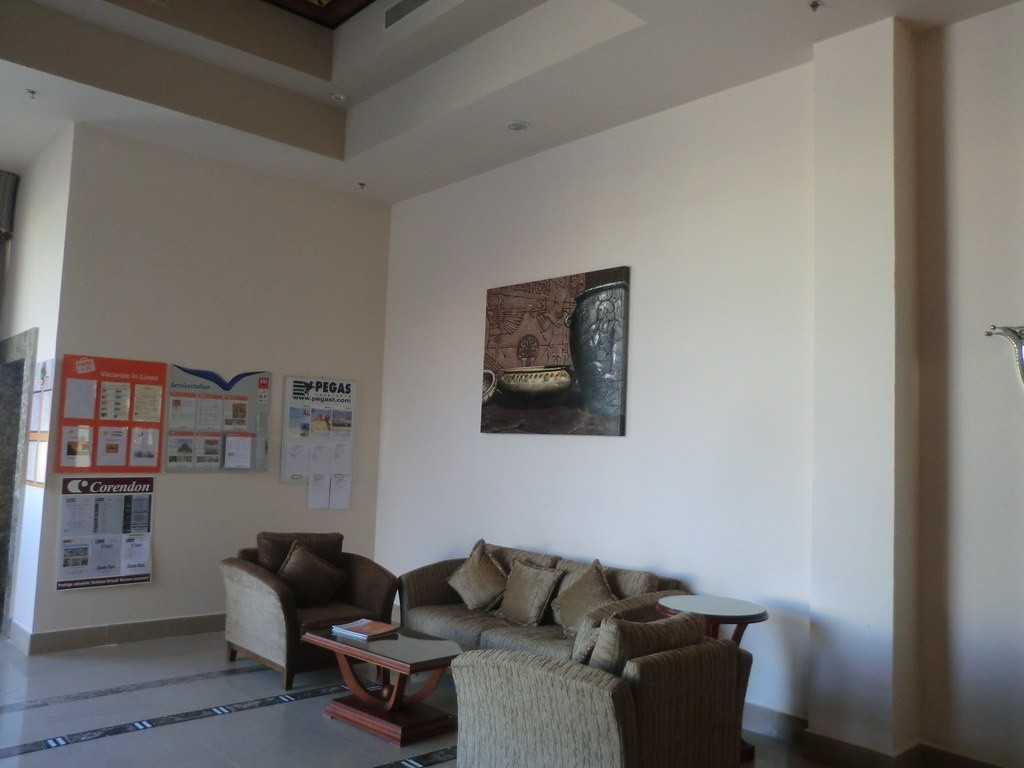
[659,596,769,762]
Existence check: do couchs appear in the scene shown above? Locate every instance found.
[397,544,752,768]
[219,547,398,689]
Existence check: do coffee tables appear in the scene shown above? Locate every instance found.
[301,622,463,747]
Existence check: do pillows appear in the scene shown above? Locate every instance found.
[278,540,347,608]
[256,531,344,577]
[551,558,618,637]
[444,540,508,611]
[495,558,564,627]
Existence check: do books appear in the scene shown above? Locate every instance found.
[331,618,400,640]
